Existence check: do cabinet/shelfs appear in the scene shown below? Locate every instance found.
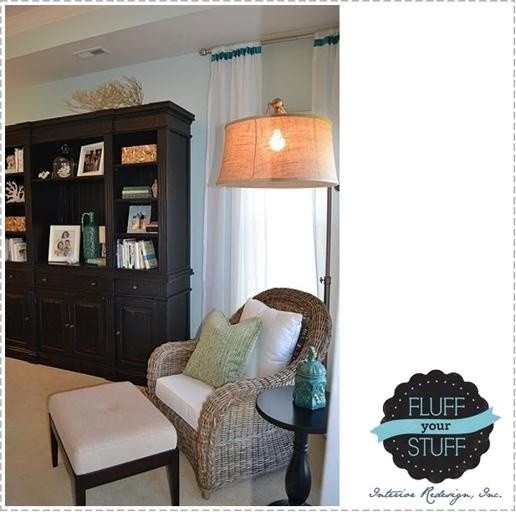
[4,96,200,390]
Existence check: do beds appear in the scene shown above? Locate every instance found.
[253,386,331,506]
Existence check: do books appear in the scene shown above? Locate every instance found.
[116,238,158,270]
[5,238,27,262]
[121,186,152,198]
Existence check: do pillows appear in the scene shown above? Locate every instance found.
[180,295,304,387]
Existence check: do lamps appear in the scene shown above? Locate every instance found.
[210,92,339,313]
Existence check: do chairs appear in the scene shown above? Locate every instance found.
[142,286,334,500]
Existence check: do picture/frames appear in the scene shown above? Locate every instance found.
[125,204,152,234]
[45,224,82,264]
[76,141,106,178]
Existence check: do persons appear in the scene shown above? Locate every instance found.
[53,231,73,257]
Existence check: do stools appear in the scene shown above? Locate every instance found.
[44,378,184,506]
